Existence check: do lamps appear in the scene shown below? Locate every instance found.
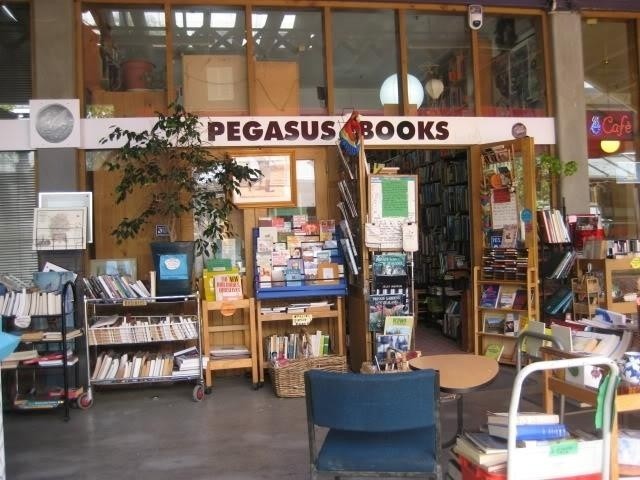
[423,13,444,99]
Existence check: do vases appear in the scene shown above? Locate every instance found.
[122,61,155,88]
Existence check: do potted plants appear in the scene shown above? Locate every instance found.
[94,90,266,296]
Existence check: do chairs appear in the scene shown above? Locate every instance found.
[301,367,445,480]
[506,355,620,480]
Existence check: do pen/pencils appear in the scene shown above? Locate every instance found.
[390,361,395,370]
[374,355,381,371]
[385,363,389,370]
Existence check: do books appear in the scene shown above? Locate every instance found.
[367,150,415,173]
[214,273,244,302]
[265,330,330,362]
[82,272,151,304]
[14,393,65,409]
[209,345,251,361]
[0,262,78,317]
[542,248,578,280]
[337,180,360,274]
[446,410,601,480]
[480,284,501,310]
[498,286,518,310]
[483,313,525,336]
[90,314,198,343]
[511,289,527,310]
[91,346,209,381]
[416,149,469,340]
[20,326,85,342]
[525,319,546,358]
[369,252,413,364]
[481,247,529,281]
[261,300,335,314]
[336,180,359,275]
[0,349,79,369]
[484,343,504,363]
[551,306,633,357]
[535,207,571,244]
[500,223,519,248]
[542,287,575,315]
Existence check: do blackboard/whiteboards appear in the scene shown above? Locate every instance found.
[368,173,419,251]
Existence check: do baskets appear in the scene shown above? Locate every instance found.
[360,346,421,374]
[269,327,346,398]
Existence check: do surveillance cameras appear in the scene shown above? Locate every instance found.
[467,4,484,31]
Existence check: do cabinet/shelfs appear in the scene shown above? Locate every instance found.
[254,296,345,382]
[198,297,260,388]
[76,289,205,412]
[367,249,419,374]
[536,204,578,320]
[539,345,640,478]
[366,148,472,342]
[0,280,80,423]
[573,255,639,315]
[472,266,540,368]
[250,224,350,300]
